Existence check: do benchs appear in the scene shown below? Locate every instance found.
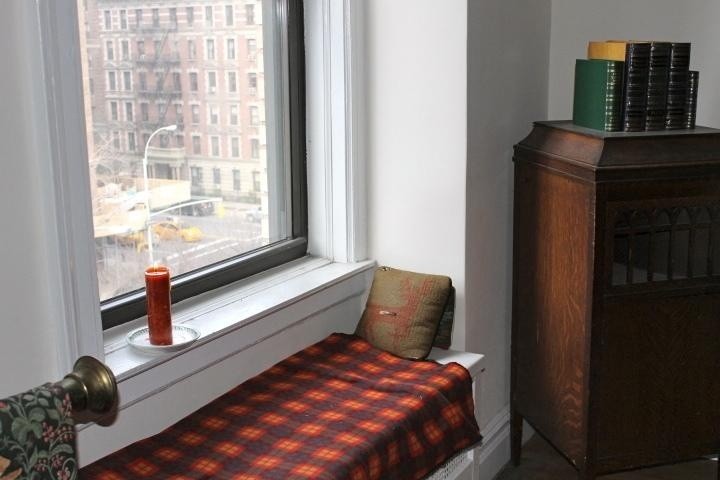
[78,331,483,480]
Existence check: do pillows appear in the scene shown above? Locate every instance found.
[353,266,454,360]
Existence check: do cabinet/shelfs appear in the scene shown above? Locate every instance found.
[511,123,718,477]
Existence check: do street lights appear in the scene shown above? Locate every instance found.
[245,206,262,221]
[142,125,177,262]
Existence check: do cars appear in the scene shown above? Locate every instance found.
[110,220,203,252]
[171,199,215,216]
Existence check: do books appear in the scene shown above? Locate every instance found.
[573,41,699,132]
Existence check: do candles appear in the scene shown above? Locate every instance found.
[145,268,173,343]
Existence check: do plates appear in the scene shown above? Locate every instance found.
[125,324,201,355]
